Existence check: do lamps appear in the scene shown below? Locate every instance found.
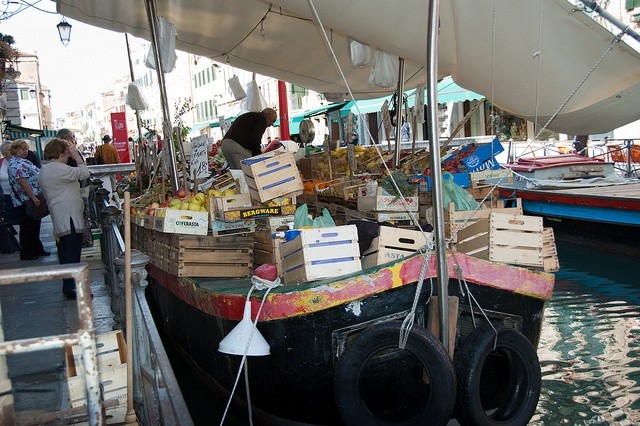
[217,301,271,358]
[57,17,72,46]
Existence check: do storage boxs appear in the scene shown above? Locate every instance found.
[469,157,500,172]
[155,232,162,269]
[278,225,361,284]
[170,235,254,278]
[207,192,256,238]
[442,136,504,172]
[343,187,358,210]
[131,214,144,227]
[330,185,344,206]
[410,173,470,190]
[359,225,431,268]
[443,199,524,238]
[315,183,331,204]
[457,213,543,270]
[130,225,155,265]
[240,150,303,203]
[144,215,154,230]
[162,234,171,274]
[543,227,559,272]
[356,185,419,212]
[152,207,209,236]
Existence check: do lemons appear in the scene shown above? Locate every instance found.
[354,146,377,163]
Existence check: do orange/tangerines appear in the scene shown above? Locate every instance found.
[297,177,340,194]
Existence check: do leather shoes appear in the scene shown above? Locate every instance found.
[66,289,94,302]
[40,249,51,256]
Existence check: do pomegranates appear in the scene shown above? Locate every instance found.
[443,144,477,173]
[409,168,431,183]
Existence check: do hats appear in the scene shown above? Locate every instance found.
[102,135,111,142]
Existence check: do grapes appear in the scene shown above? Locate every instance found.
[321,181,368,199]
[138,192,160,208]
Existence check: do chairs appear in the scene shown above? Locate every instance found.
[629,145,640,173]
[608,145,628,168]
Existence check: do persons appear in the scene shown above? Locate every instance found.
[0,141,22,255]
[221,108,277,170]
[7,139,51,260]
[56,128,85,167]
[38,139,93,300]
[94,134,120,164]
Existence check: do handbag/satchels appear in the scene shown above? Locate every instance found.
[21,193,50,222]
[95,145,105,165]
[82,197,94,247]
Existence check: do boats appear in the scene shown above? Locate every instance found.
[488,159,639,229]
[144,248,556,425]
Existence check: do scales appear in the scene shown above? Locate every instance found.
[299,120,315,157]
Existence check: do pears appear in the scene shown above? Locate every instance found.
[367,154,391,168]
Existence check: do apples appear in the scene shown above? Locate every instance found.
[194,189,238,204]
[155,198,207,220]
[145,188,188,213]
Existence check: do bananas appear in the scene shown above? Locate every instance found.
[323,147,348,158]
